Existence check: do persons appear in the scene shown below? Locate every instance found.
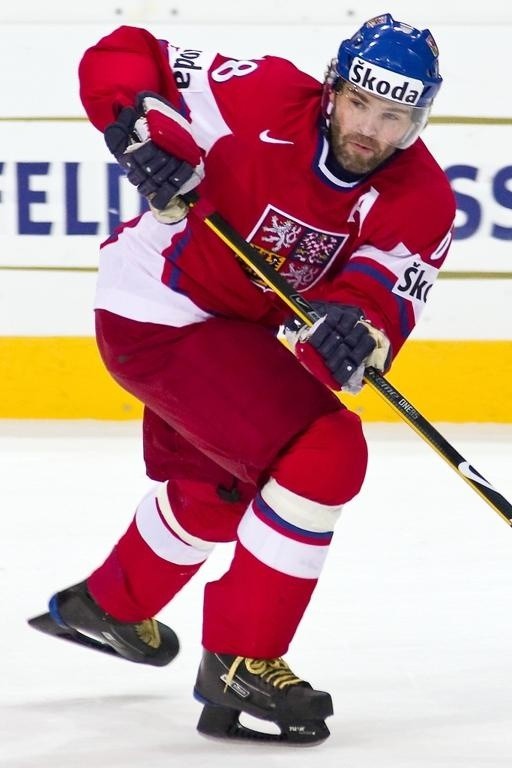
[46,12,460,724]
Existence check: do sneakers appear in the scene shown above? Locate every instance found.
[194,651,333,721]
[49,582,178,666]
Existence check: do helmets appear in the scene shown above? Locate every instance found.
[322,13,443,151]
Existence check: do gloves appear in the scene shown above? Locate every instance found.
[285,301,376,395]
[121,93,206,209]
